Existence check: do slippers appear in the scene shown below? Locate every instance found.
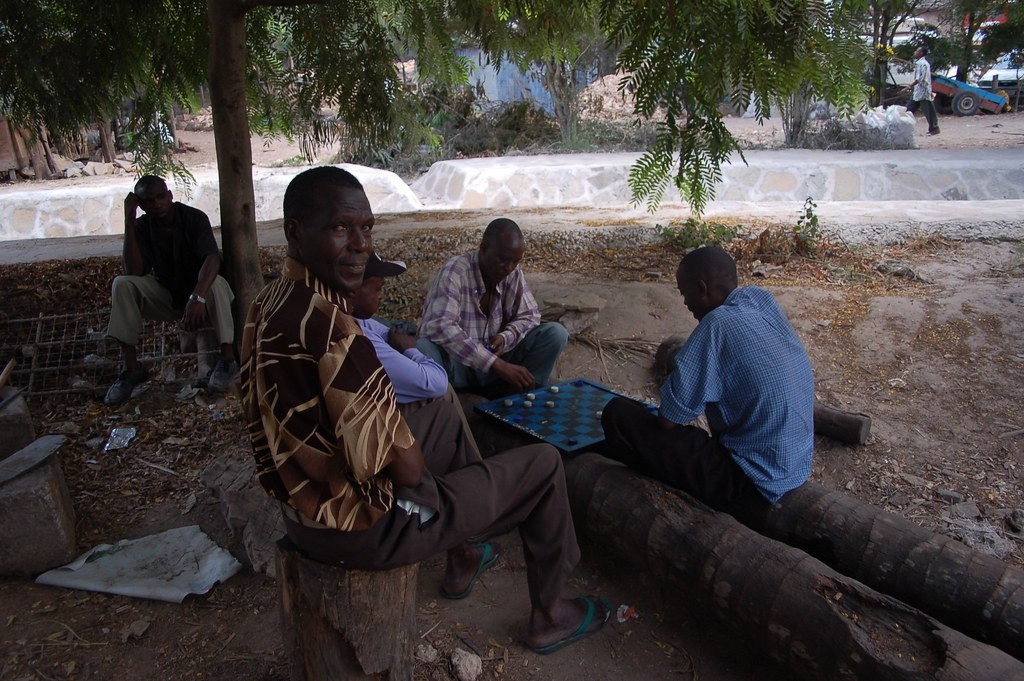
[532,592,610,655]
[443,540,500,599]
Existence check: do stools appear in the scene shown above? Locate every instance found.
[0,383,35,463]
[275,532,418,681]
[0,451,77,578]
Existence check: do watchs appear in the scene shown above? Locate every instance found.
[188,293,206,304]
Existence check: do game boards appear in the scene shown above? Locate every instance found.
[476,376,660,458]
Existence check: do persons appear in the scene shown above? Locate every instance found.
[104,172,235,405]
[346,248,484,477]
[900,46,940,135]
[239,165,612,655]
[600,243,814,518]
[418,219,568,394]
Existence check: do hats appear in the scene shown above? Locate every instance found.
[365,249,407,278]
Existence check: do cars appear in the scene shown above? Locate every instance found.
[816,16,1024,103]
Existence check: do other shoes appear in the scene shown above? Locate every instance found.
[207,358,240,391]
[104,360,148,404]
[927,127,940,136]
